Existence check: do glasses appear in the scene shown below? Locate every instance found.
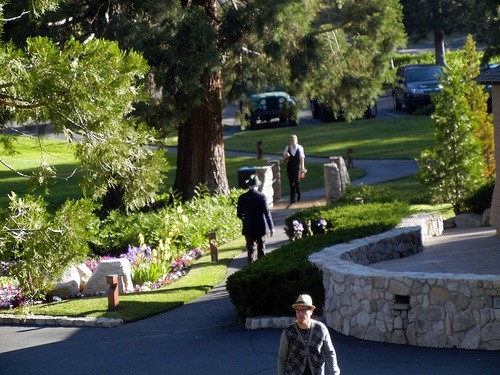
[295,309,310,312]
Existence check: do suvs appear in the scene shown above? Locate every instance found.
[391,63,446,114]
[238,77,297,129]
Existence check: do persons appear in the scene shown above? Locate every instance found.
[283,134,307,203]
[236,173,275,262]
[277,293,341,375]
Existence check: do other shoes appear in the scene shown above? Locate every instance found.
[298,195,301,201]
[290,199,295,203]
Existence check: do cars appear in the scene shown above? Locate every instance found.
[308,92,378,120]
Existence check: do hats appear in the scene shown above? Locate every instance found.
[245,174,262,186]
[292,294,316,310]
